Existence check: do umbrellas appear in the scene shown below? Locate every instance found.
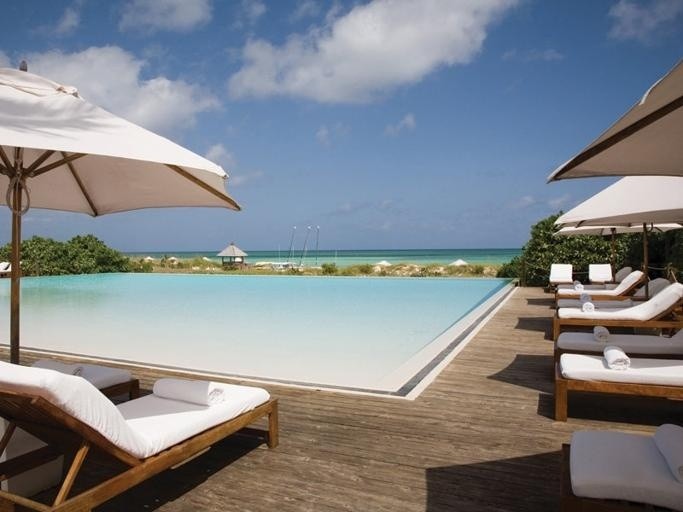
[552,227,683,284]
[0,58,241,365]
[541,63,683,188]
[551,172,683,300]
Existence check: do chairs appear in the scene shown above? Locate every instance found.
[569,423,682,512]
[549,263,683,422]
